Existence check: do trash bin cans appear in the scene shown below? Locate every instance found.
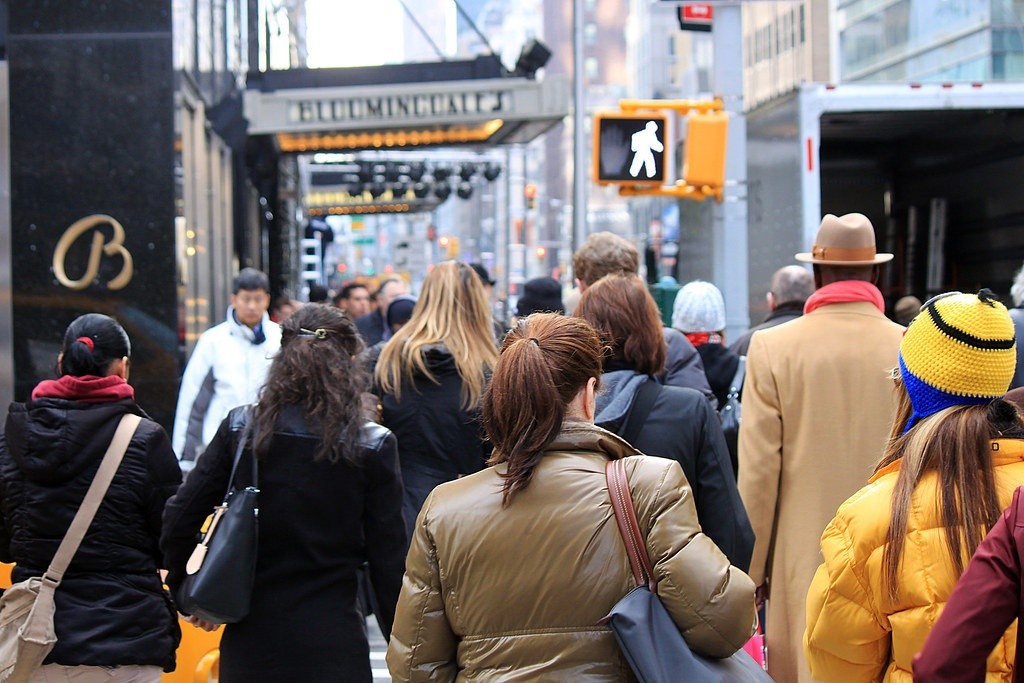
[650,283,679,327]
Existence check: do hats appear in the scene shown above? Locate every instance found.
[895,288,1018,437]
[387,299,417,327]
[794,213,894,266]
[671,281,726,334]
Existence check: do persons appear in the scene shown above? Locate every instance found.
[0,212,1024,683]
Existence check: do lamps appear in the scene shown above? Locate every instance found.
[510,39,551,80]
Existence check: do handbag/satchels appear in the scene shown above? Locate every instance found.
[716,354,747,484]
[0,576,58,683]
[165,487,264,624]
[595,456,776,683]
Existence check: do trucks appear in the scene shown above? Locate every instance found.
[645,79,1024,362]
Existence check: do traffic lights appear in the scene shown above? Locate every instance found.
[526,182,535,205]
[593,110,668,185]
